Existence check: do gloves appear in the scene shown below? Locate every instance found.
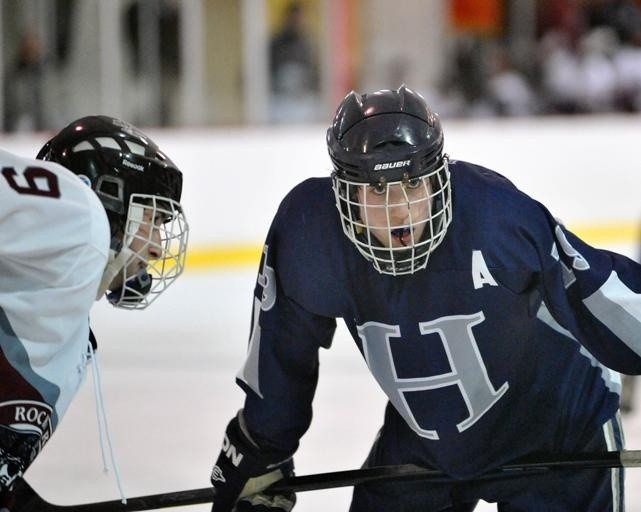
[211,407,295,511]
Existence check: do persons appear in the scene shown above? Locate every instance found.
[209,86,640,511]
[0,1,640,133]
[2,110,192,512]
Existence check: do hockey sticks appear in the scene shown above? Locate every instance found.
[6,450,640,512]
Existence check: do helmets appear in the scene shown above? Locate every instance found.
[36,115,189,310]
[325,82,454,277]
